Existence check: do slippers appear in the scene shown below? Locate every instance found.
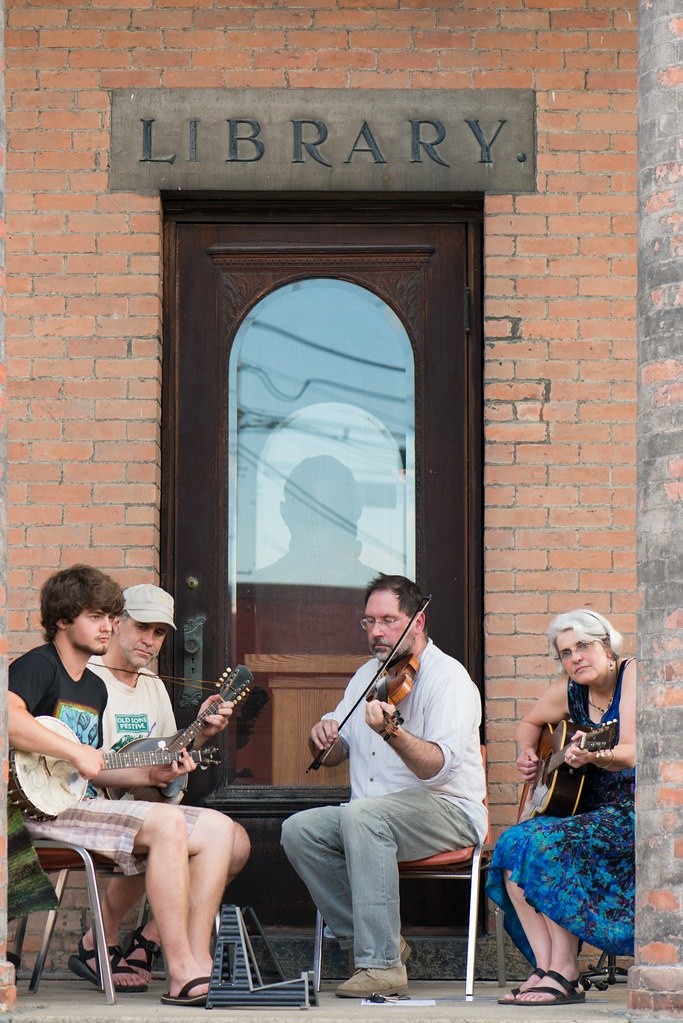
[161,976,213,1006]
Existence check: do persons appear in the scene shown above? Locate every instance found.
[83,585,252,994]
[3,561,240,1004]
[278,570,490,1001]
[489,605,637,1005]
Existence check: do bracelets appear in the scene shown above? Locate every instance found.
[596,750,615,767]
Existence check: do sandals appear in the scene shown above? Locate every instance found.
[122,924,162,972]
[497,968,586,1005]
[66,932,148,992]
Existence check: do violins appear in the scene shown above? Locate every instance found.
[365,653,418,739]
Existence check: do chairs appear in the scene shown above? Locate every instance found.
[7,739,225,1007]
[313,746,507,1002]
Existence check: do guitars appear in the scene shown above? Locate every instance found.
[107,664,252,803]
[9,715,219,822]
[516,718,621,825]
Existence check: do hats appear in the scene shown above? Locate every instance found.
[121,583,177,631]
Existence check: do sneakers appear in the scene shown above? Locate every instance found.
[335,932,412,997]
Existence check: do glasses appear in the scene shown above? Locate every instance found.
[556,639,600,661]
[360,613,408,631]
